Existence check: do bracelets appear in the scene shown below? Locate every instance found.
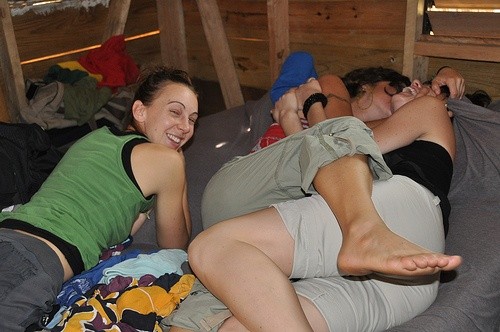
[302,93,328,120]
[436,66,451,76]
[145,212,150,220]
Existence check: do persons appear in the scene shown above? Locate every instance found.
[159,64,466,332]
[0,65,199,332]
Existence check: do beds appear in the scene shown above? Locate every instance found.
[133,93,500,332]
